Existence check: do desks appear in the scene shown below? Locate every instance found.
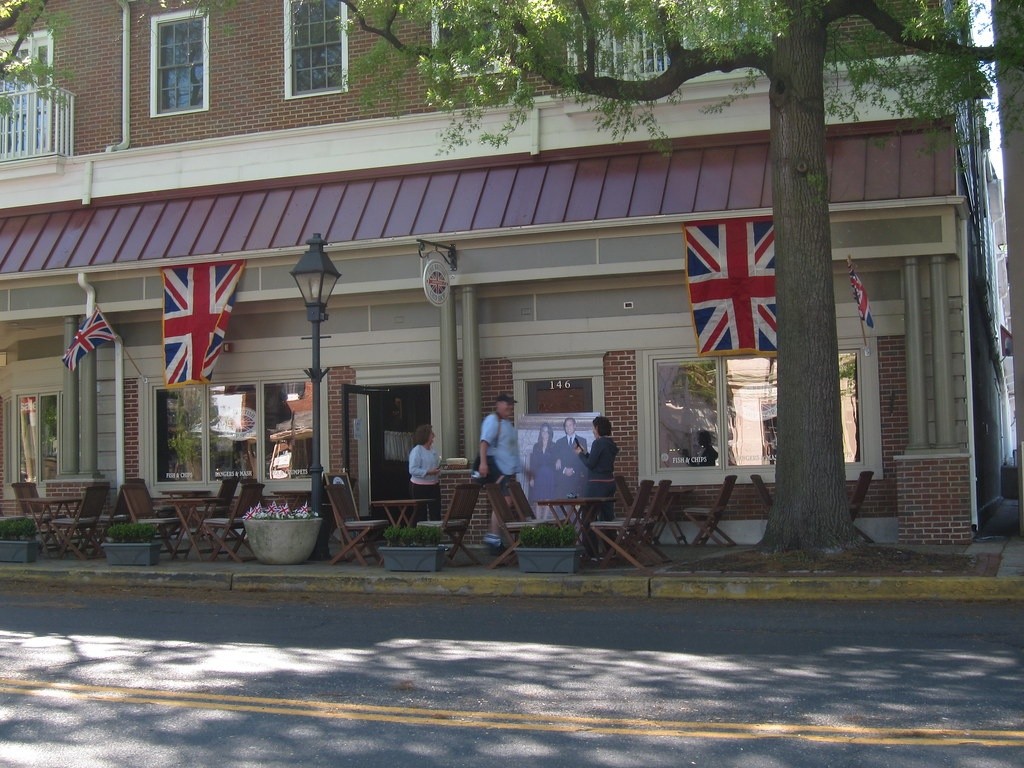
[537,497,616,563]
[18,496,81,559]
[166,496,220,562]
[158,490,213,549]
[370,498,438,568]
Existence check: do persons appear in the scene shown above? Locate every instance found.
[409,424,448,551]
[478,394,524,557]
[523,422,555,518]
[553,417,588,518]
[575,415,619,562]
[678,429,717,467]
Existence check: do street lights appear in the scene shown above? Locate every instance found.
[290,231,341,561]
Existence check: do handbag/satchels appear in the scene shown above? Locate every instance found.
[472,454,495,482]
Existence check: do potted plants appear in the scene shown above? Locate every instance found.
[379,525,448,571]
[516,525,585,574]
[0,517,41,564]
[103,526,162,568]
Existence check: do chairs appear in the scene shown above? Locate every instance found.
[13,470,875,573]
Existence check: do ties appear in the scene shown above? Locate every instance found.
[569,437,572,446]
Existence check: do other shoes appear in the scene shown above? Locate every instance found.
[490,544,515,556]
[579,551,617,561]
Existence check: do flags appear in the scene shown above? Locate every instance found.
[160,259,247,389]
[682,217,778,360]
[846,259,875,329]
[61,309,118,371]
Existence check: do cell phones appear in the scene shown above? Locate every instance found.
[574,438,583,451]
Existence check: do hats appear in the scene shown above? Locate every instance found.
[496,393,518,404]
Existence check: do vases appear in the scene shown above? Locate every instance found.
[241,515,321,565]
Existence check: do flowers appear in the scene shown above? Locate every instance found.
[244,503,318,520]
